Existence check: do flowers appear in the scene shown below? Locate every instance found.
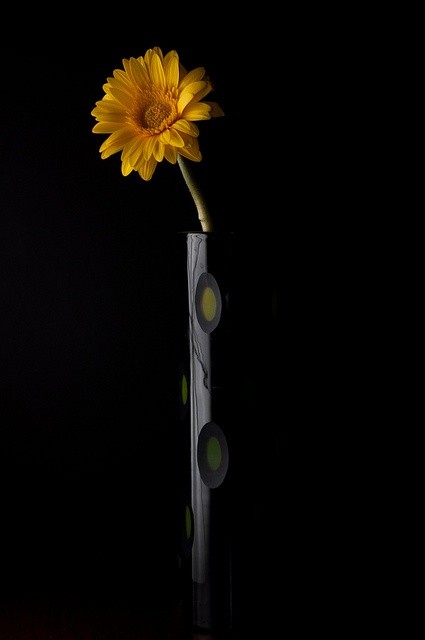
[90,44,225,234]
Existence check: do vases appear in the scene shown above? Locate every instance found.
[175,231,241,629]
